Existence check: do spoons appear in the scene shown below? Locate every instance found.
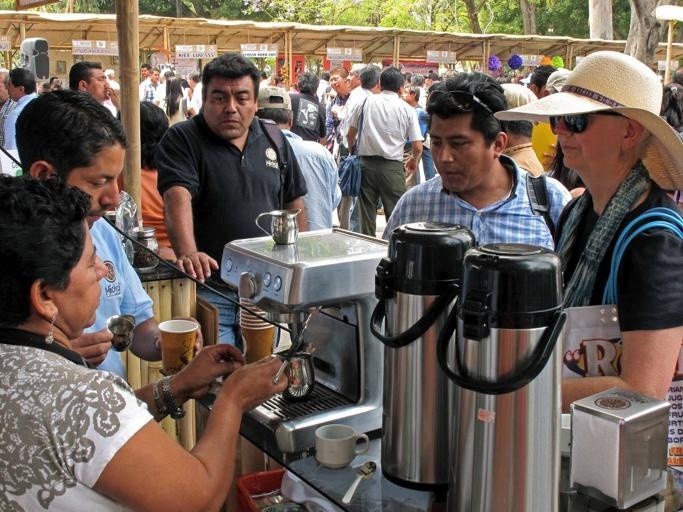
[338,462,377,504]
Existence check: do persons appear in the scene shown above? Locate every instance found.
[15,90,203,374]
[494,52,682,466]
[158,53,308,345]
[0,50,681,237]
[381,71,573,253]
[0,173,288,511]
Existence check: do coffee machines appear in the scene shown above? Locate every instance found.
[209,222,392,454]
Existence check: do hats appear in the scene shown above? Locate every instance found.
[493,51,683,191]
[257,85,292,110]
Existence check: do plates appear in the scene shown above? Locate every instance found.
[257,501,307,511]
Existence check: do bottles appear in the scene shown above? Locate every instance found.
[127,226,160,271]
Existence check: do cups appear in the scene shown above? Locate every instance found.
[156,319,198,377]
[557,409,572,455]
[239,296,275,365]
[253,207,302,246]
[105,313,137,353]
[312,424,369,470]
[274,350,315,401]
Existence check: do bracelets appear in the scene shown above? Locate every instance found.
[161,373,185,419]
[151,380,167,415]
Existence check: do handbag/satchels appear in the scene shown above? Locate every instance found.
[338,155,361,197]
[562,207,683,472]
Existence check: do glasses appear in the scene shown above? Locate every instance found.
[426,90,493,111]
[550,112,629,133]
